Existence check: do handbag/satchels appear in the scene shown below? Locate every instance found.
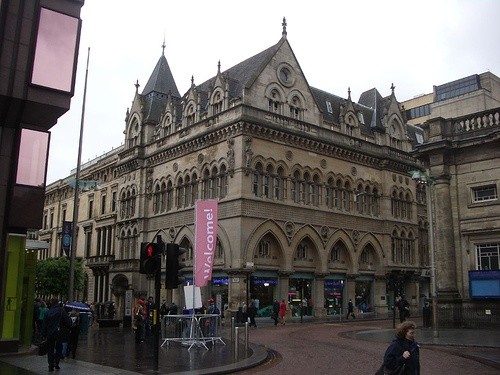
[375,340,405,375]
[37,338,46,355]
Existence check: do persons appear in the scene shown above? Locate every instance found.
[208,299,220,335]
[41,297,72,372]
[242,302,247,312]
[346,299,355,320]
[272,298,283,326]
[248,303,257,327]
[34,297,178,359]
[302,297,313,316]
[383,321,421,375]
[396,295,409,322]
[254,297,260,310]
[279,299,287,325]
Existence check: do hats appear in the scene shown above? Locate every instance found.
[208,298,214,304]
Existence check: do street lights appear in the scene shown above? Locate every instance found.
[412,172,440,337]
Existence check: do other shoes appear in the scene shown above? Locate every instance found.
[48,366,54,371]
[54,363,60,369]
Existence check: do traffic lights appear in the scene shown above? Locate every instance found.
[139,241,158,274]
[165,241,187,289]
